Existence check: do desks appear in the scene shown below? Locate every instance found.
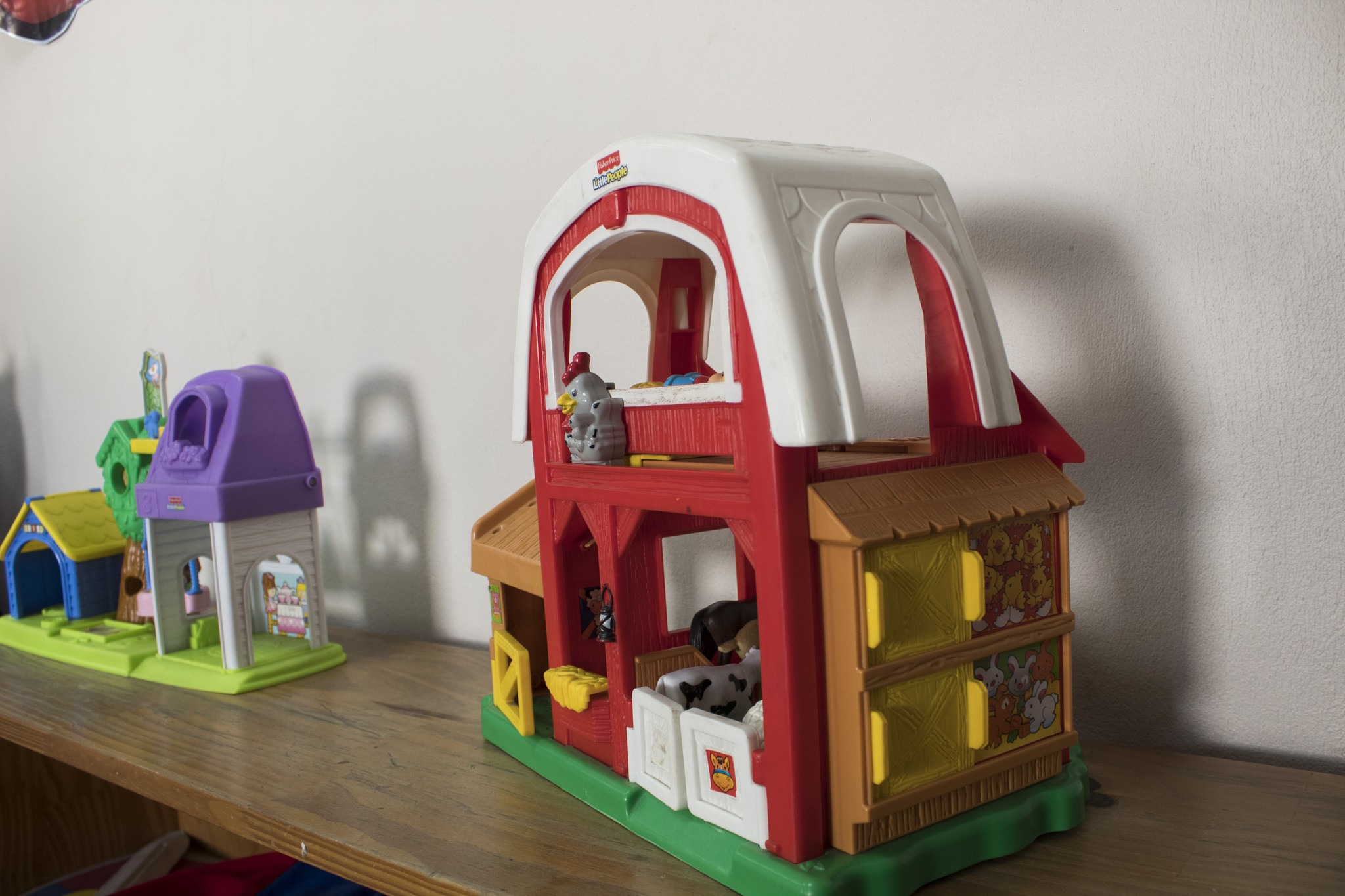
[1,625,1345,896]
